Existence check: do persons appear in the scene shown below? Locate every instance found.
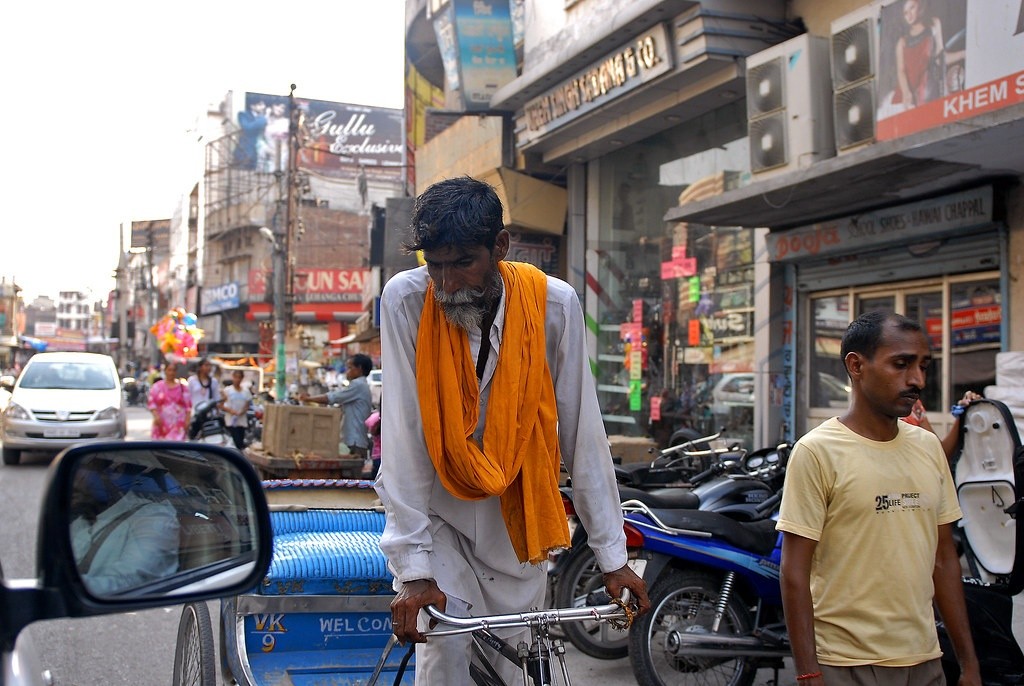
[899,390,987,467]
[185,359,226,430]
[773,306,984,686]
[368,173,653,685]
[231,95,293,177]
[362,394,388,481]
[221,370,254,452]
[145,362,189,443]
[877,1,946,123]
[301,351,369,465]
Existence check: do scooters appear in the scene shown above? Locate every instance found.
[117,357,157,408]
[187,399,241,454]
[242,391,331,450]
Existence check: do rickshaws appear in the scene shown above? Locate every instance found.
[172,479,636,686]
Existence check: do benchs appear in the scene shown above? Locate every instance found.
[240,504,417,650]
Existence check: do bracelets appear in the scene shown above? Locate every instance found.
[607,597,633,632]
[795,671,824,680]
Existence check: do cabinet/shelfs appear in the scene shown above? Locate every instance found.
[706,263,754,348]
[597,322,648,426]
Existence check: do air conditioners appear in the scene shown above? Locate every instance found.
[745,32,832,183]
[828,0,896,156]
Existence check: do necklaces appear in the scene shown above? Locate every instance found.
[197,371,213,399]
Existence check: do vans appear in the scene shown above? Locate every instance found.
[704,372,850,432]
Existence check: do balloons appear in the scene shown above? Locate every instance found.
[150,306,205,365]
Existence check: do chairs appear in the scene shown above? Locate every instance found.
[39,368,60,384]
[83,369,108,386]
[166,496,238,577]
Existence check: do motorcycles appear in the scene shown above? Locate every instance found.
[619,499,799,686]
[535,424,751,642]
[557,444,795,659]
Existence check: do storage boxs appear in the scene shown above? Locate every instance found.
[261,401,342,460]
[607,432,661,466]
[707,436,746,454]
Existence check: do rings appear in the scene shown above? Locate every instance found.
[392,622,399,626]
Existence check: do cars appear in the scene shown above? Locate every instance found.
[0,352,136,466]
